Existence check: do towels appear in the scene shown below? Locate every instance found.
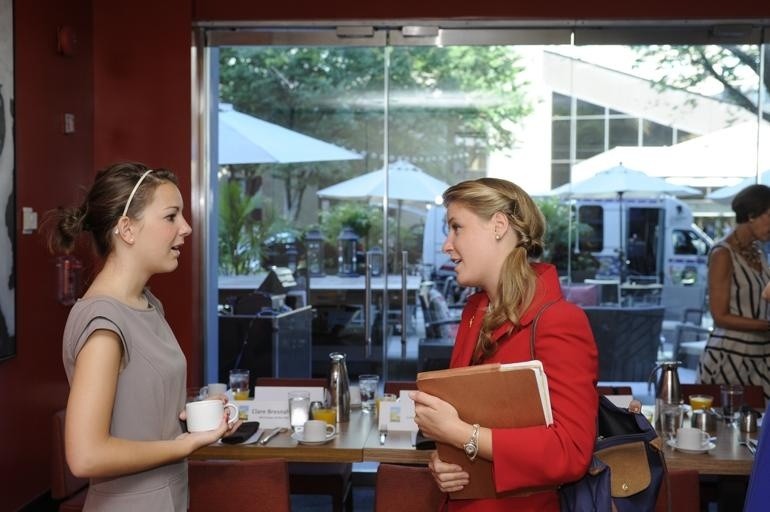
[218,266,312,398]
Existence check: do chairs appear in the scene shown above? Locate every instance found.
[184,230,769,511]
[49,405,90,512]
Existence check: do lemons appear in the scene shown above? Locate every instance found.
[648,358,685,406]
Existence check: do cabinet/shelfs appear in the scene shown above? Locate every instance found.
[325,351,352,422]
[311,401,323,418]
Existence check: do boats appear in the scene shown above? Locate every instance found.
[217,303,315,379]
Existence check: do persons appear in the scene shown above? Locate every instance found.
[410,179,599,512]
[39,161,243,512]
[743,281,770,512]
[697,184,770,408]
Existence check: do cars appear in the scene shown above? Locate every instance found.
[185,400,238,433]
[359,374,378,414]
[676,427,712,448]
[229,369,250,400]
[375,392,398,415]
[208,383,226,397]
[313,409,336,424]
[304,419,334,440]
[288,390,310,430]
[657,383,763,435]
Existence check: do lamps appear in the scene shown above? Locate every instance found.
[49,405,90,512]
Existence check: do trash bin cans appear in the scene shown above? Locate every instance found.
[464,423,480,461]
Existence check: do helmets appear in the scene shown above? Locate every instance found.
[291,431,335,444]
[665,438,718,453]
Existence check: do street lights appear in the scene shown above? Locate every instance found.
[529,299,665,511]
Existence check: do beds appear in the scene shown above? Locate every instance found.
[219,103,365,165]
[316,160,451,274]
[551,163,701,283]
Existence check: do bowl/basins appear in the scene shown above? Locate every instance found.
[735,231,761,265]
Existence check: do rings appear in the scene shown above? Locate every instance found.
[380,431,387,444]
